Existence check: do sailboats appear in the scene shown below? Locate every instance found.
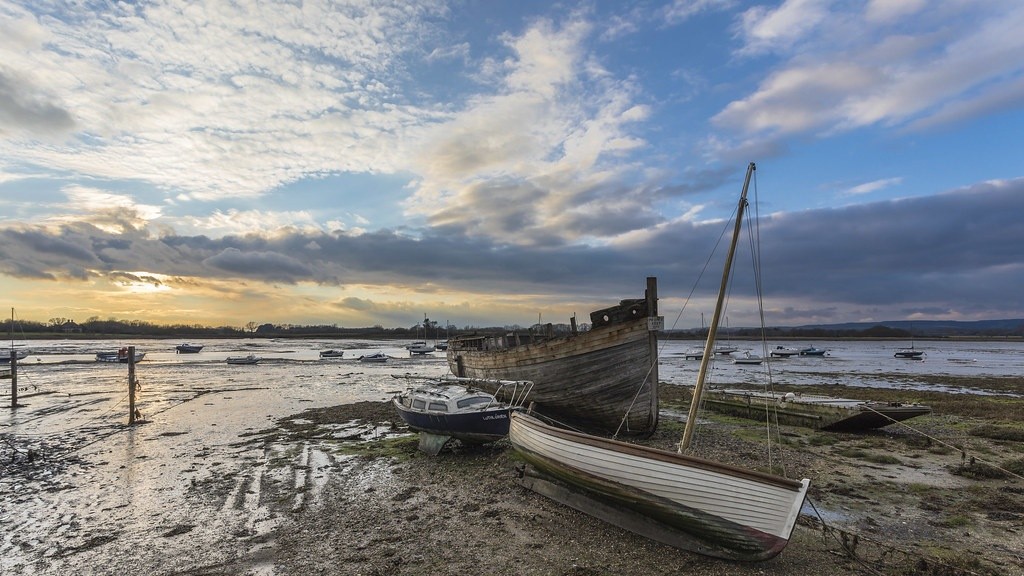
[713,316,737,354]
[0,307,30,361]
[894,320,925,360]
[507,161,811,563]
[405,312,448,353]
[685,313,704,357]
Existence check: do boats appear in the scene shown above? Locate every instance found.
[391,373,535,444]
[94,349,146,364]
[689,385,933,432]
[225,356,260,364]
[770,345,799,357]
[357,352,389,362]
[446,276,661,442]
[319,349,344,358]
[176,343,204,353]
[801,344,826,355]
[733,355,763,364]
